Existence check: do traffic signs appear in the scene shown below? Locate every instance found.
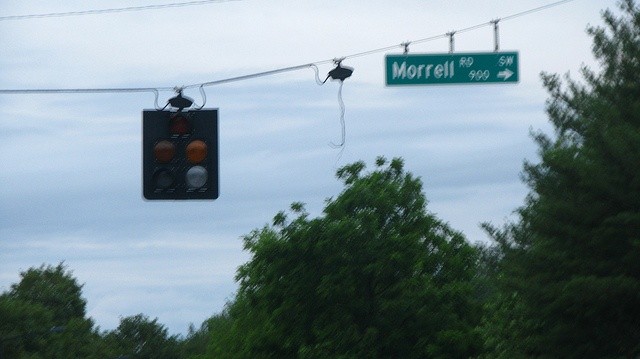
[384,52,519,85]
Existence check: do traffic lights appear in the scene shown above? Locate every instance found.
[142,109,218,199]
